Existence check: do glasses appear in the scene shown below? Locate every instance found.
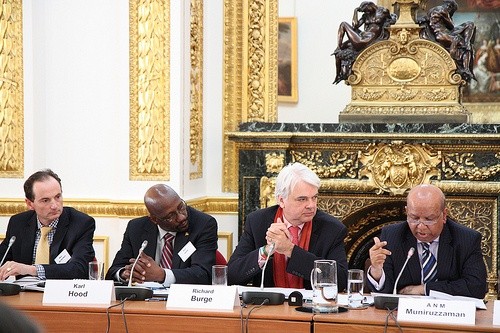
[405,208,444,226]
[156,199,187,224]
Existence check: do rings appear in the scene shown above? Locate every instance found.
[269,238,272,242]
[7,268,11,271]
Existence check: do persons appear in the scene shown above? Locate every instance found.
[330,1,391,85]
[104,183,218,288]
[225,161,349,294]
[428,0,477,81]
[364,183,487,299]
[0,169,95,281]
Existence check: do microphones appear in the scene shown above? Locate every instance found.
[373,247,415,310]
[115,240,153,301]
[241,242,286,305]
[0,235,21,296]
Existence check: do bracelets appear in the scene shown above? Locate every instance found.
[260,246,271,261]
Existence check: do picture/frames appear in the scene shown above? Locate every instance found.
[411,0,500,102]
[277,17,298,103]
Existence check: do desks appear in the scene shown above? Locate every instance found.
[0,291,499,333]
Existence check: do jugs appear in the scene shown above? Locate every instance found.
[310,260,339,313]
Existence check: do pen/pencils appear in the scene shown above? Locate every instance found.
[146,286,169,289]
[145,298,167,302]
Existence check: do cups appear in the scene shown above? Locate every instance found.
[212,265,228,286]
[347,269,364,308]
[88,261,104,280]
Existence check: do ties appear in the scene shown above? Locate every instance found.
[160,234,175,269]
[288,226,300,288]
[34,226,53,265]
[421,240,437,285]
